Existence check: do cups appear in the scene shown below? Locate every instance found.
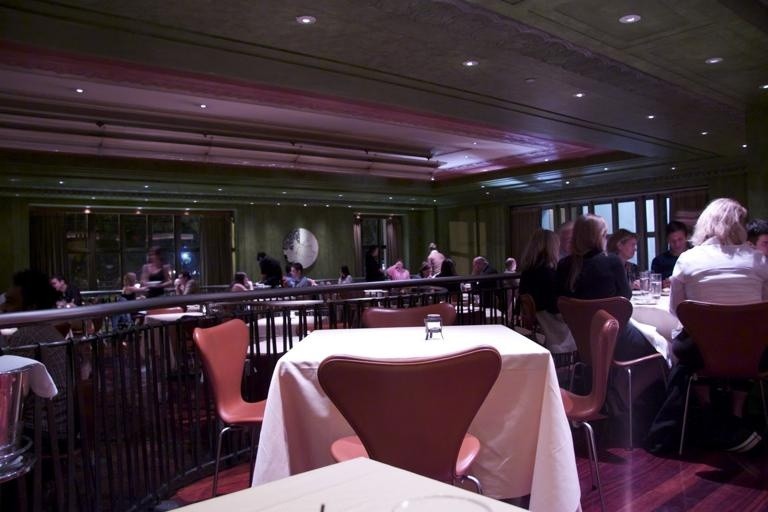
[391,494,492,512]
[639,269,662,299]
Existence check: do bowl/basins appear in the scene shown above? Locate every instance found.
[146,281,162,287]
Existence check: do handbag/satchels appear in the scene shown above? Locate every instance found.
[639,379,707,463]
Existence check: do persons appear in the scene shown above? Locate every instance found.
[0,268,75,475]
[608,228,641,291]
[554,211,658,424]
[121,245,196,300]
[365,242,520,308]
[51,275,82,307]
[650,220,691,289]
[231,252,312,292]
[338,265,352,284]
[513,227,561,315]
[555,222,575,261]
[668,195,768,433]
[746,217,768,256]
